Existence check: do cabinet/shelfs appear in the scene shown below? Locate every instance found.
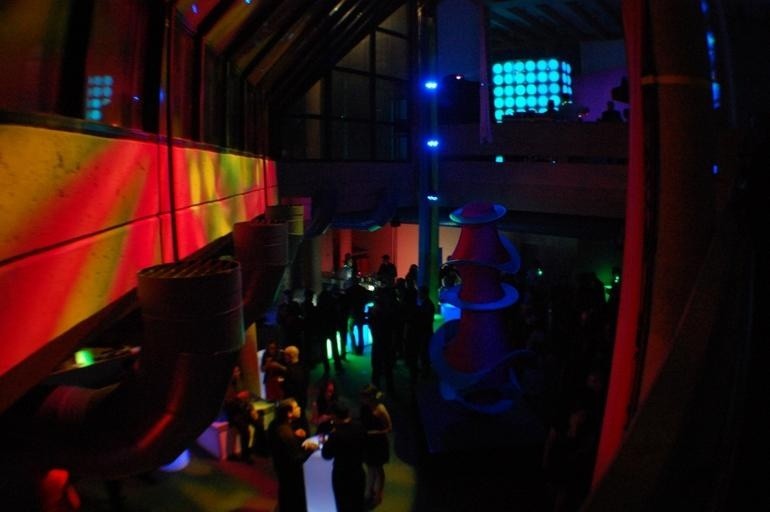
[195,397,277,463]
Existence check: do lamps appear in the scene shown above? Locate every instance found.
[265,199,305,238]
[231,0,291,271]
[134,0,248,359]
